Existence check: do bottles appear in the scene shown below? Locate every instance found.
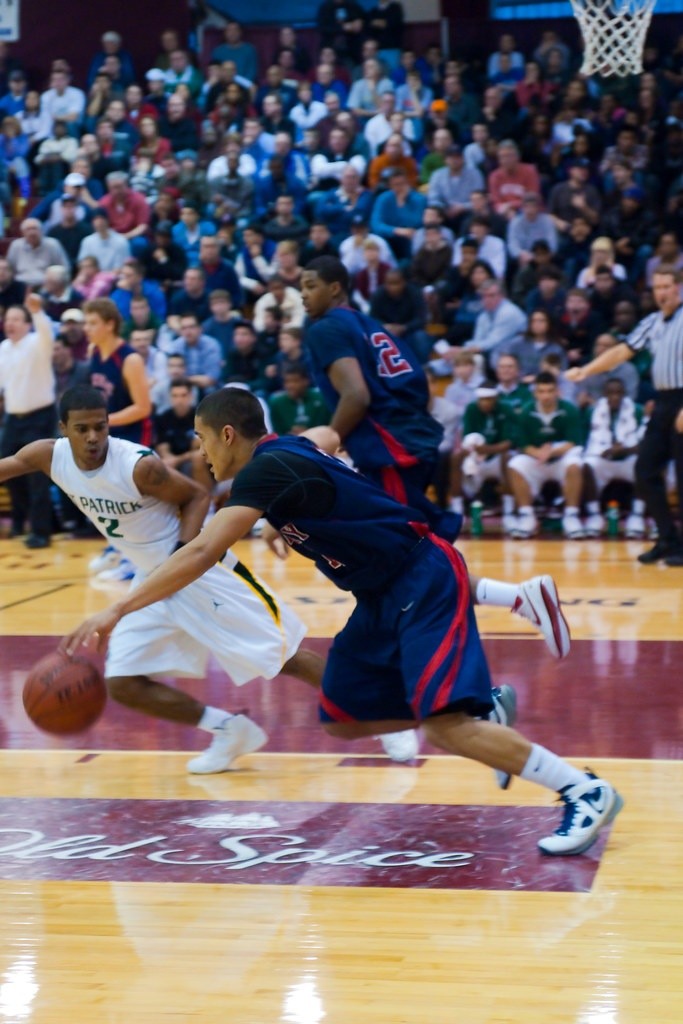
[472,500,483,533]
[608,500,619,536]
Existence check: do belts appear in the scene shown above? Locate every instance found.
[9,405,50,419]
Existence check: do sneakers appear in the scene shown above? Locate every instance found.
[536,766,622,855]
[375,730,421,764]
[475,686,516,788]
[184,711,267,775]
[511,576,570,662]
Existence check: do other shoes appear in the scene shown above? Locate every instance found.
[25,534,52,550]
[639,540,682,564]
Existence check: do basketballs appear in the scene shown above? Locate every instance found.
[22,652,105,740]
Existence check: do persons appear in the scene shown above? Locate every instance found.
[0,1,683,573]
[56,386,623,855]
[1,386,418,776]
[298,255,571,658]
